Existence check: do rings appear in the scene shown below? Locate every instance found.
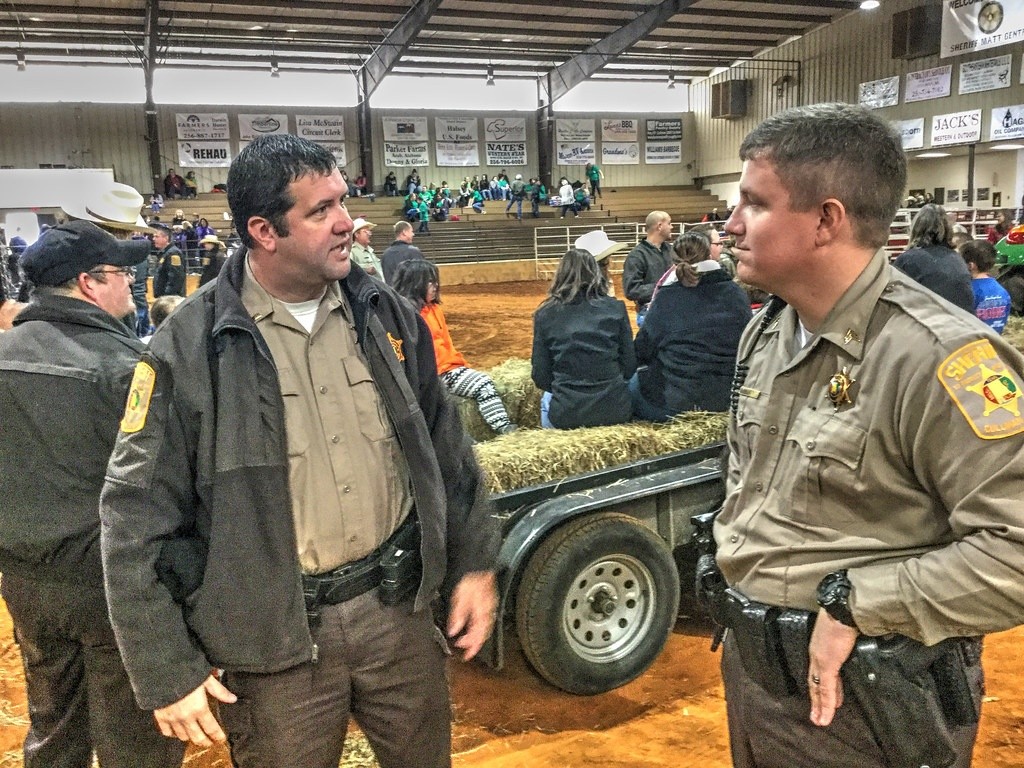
[812,676,820,684]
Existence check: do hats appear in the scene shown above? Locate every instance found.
[197,234,227,250]
[61,182,158,235]
[574,229,629,262]
[352,218,378,235]
[20,219,152,287]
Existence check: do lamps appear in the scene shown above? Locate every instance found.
[487,47,495,86]
[269,36,280,77]
[669,59,676,88]
[16,20,26,72]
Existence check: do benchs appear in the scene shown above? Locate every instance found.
[142,184,726,262]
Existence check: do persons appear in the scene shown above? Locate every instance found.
[575,229,629,297]
[946,196,1024,253]
[707,205,735,221]
[891,203,974,315]
[98,135,502,768]
[149,168,198,214]
[0,219,189,768]
[622,210,674,328]
[958,239,1012,335]
[900,192,938,208]
[531,249,639,429]
[392,259,519,444]
[691,101,1024,768]
[350,218,385,283]
[381,221,426,286]
[647,223,724,311]
[0,227,34,331]
[60,182,242,344]
[340,163,604,232]
[629,233,753,423]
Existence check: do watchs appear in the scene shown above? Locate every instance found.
[816,568,857,627]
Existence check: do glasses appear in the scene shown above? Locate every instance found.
[710,241,725,248]
[88,264,138,277]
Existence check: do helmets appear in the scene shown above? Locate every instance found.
[515,174,523,180]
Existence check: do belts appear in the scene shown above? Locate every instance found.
[302,517,417,607]
